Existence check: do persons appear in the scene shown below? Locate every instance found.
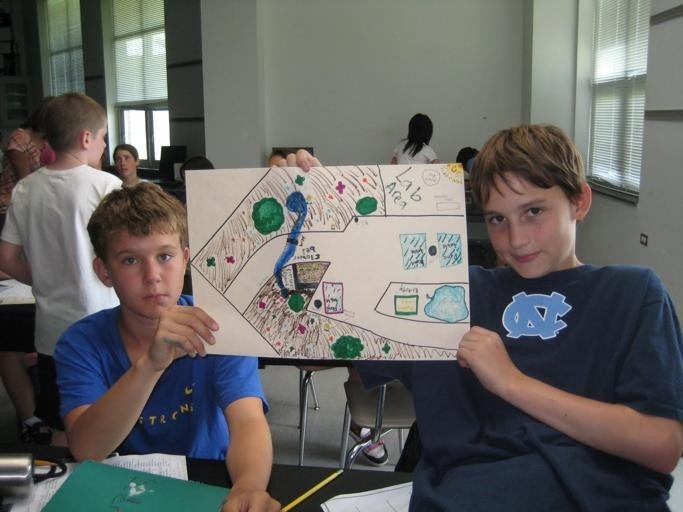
[391,114,439,165]
[281,119,683,512]
[114,145,147,187]
[0,93,122,456]
[53,179,281,512]
[179,157,214,187]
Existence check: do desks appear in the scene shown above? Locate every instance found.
[0,444,414,511]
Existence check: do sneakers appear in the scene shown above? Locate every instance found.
[349,423,389,466]
[21,421,52,445]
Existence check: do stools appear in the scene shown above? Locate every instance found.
[339,381,416,471]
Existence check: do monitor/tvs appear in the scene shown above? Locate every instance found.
[272,147,313,159]
[159,145,187,177]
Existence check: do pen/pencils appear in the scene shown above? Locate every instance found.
[281,469,344,512]
